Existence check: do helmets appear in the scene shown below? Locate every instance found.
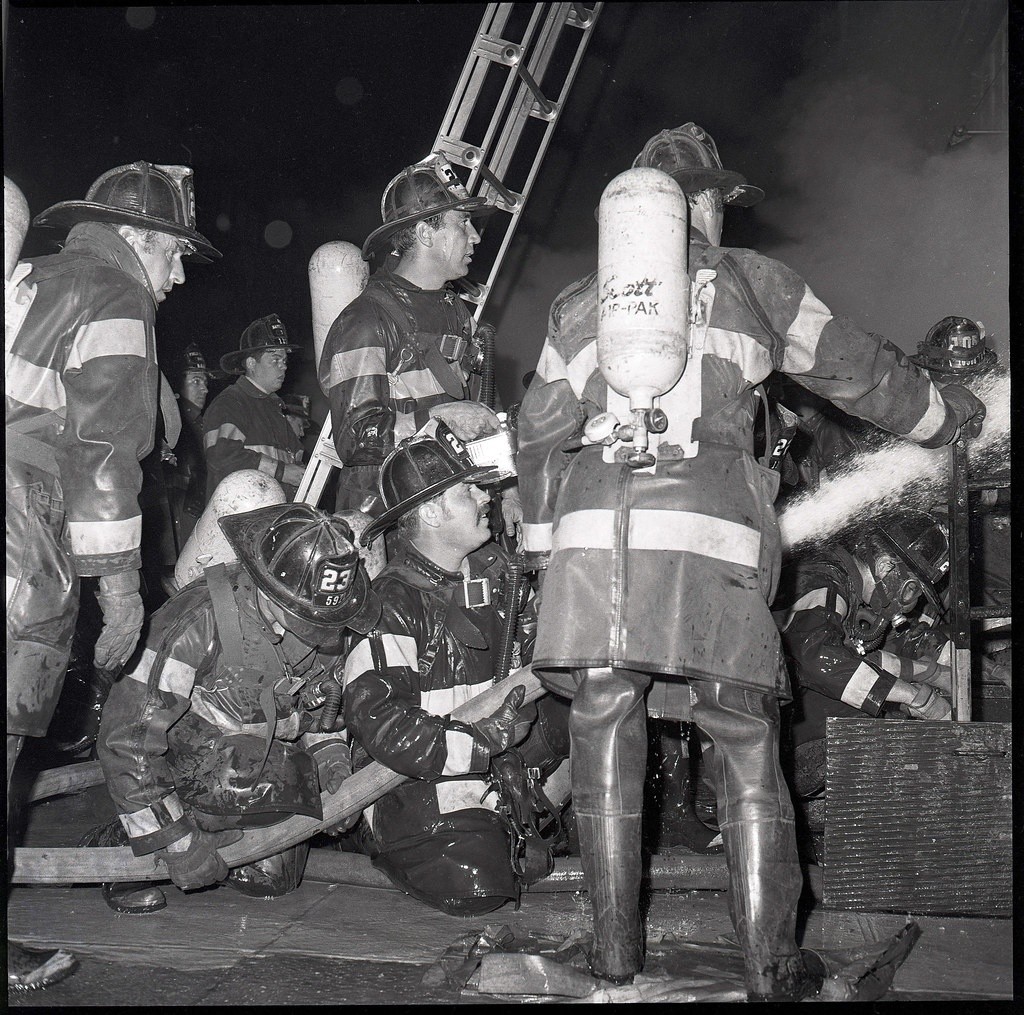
[870,508,950,615]
[179,343,218,374]
[280,393,316,428]
[358,436,498,549]
[362,151,487,261]
[32,160,223,264]
[593,123,764,224]
[217,502,382,648]
[907,315,997,374]
[220,313,304,374]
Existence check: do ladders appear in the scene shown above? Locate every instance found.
[292,0,606,524]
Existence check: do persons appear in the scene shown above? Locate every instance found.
[516,119,996,1013]
[1,145,570,985]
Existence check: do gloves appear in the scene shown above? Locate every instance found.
[475,685,537,757]
[313,743,361,836]
[154,829,245,891]
[899,682,953,720]
[939,384,986,440]
[913,663,952,697]
[93,569,144,672]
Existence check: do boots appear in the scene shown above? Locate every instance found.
[651,755,724,856]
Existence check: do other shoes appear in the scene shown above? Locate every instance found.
[584,955,636,985]
[746,948,827,1002]
[86,820,167,915]
[8,940,80,991]
